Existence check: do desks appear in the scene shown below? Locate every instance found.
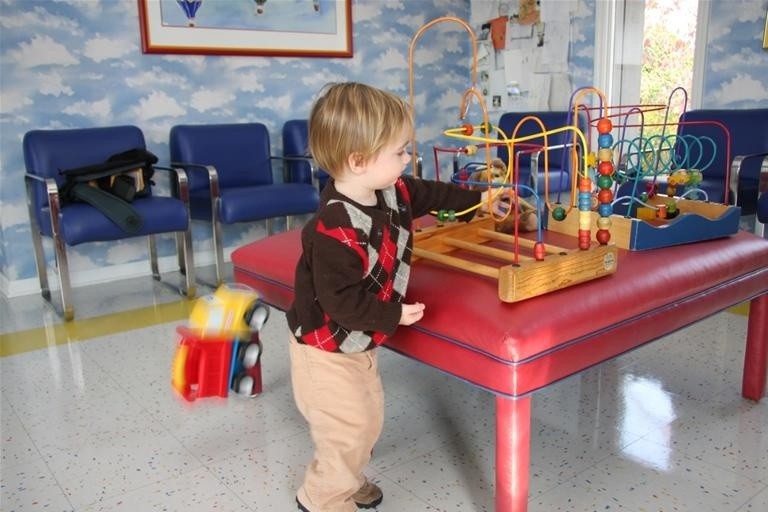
[228,211,768,511]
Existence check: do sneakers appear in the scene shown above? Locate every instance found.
[350,481,383,509]
[296,495,310,512]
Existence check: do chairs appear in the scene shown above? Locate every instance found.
[167,122,330,288]
[453,111,585,205]
[616,108,768,219]
[22,125,199,322]
[754,156,767,238]
[283,120,423,192]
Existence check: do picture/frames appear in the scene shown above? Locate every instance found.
[138,0,353,57]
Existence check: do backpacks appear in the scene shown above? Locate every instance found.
[53,148,157,233]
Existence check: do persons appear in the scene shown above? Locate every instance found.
[285,80,513,512]
